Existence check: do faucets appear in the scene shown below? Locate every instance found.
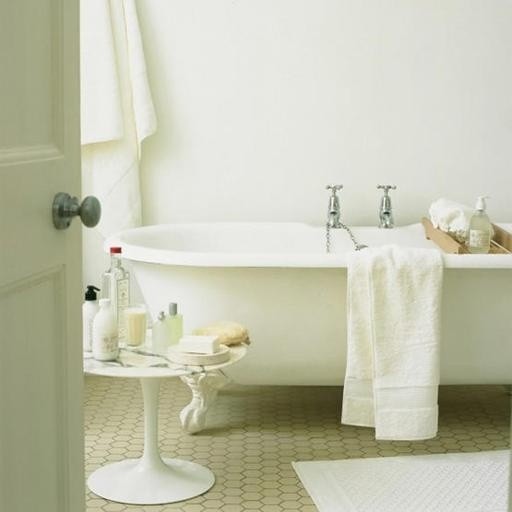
[324,184,344,229]
[376,183,397,229]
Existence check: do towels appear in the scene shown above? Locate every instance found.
[339,243,445,441]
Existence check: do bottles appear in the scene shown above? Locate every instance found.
[468,195,490,254]
[164,304,183,345]
[92,298,119,360]
[104,246,130,309]
[152,311,171,349]
[82,285,101,351]
[128,305,147,346]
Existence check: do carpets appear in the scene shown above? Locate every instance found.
[292,449,512,511]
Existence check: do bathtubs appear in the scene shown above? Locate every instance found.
[108,221,512,435]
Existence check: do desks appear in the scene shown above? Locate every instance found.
[85,328,248,507]
